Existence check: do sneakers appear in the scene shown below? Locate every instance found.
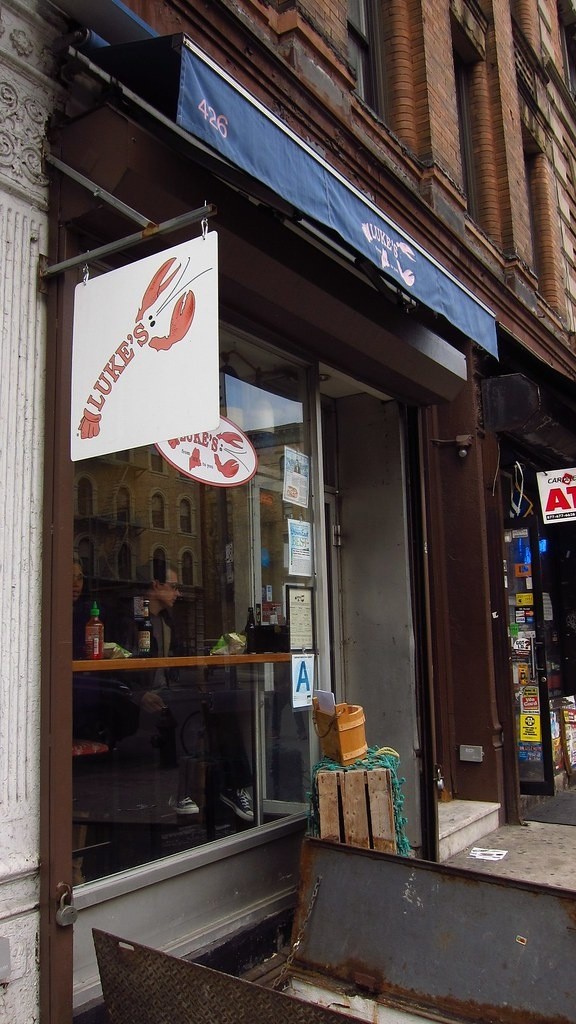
[166,790,200,815]
[218,785,255,821]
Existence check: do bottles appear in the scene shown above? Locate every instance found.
[269,607,278,625]
[245,607,256,653]
[85,601,104,660]
[138,600,154,656]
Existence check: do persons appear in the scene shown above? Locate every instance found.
[70,556,141,852]
[112,557,259,823]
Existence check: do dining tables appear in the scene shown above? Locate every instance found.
[71,653,292,876]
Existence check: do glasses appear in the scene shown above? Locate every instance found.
[159,580,184,592]
[73,573,86,581]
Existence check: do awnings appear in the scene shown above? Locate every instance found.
[74,26,502,363]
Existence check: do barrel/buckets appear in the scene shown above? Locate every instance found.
[314,698,366,766]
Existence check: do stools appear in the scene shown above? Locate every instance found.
[69,739,119,879]
